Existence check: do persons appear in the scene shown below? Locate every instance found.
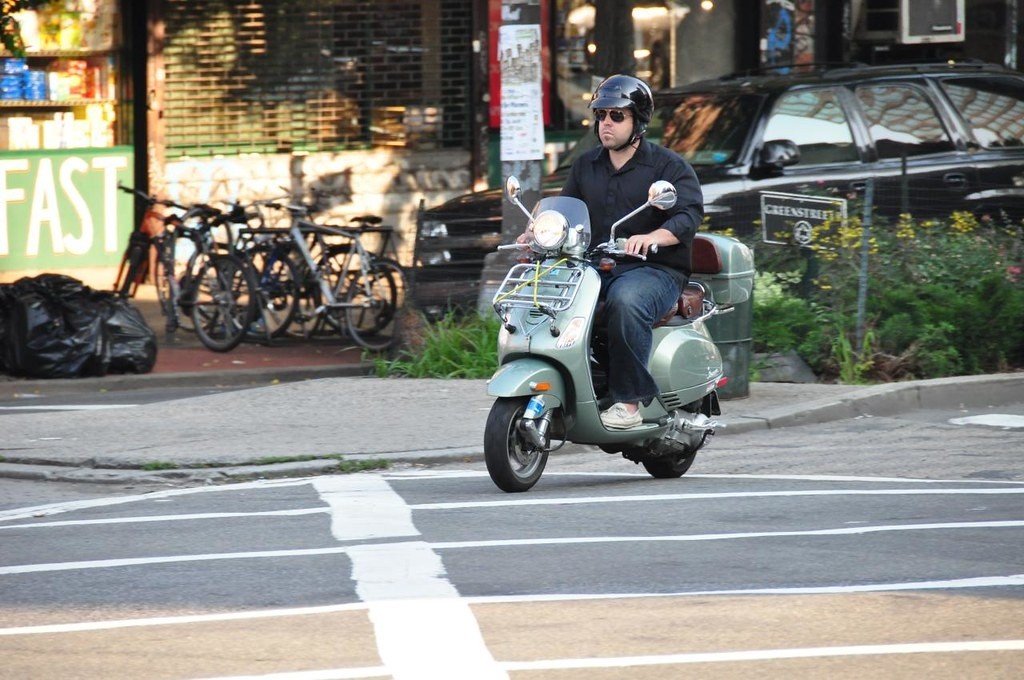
[516,74,704,430]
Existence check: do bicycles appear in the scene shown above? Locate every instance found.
[112,182,408,354]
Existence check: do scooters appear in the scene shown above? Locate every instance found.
[483,176,729,496]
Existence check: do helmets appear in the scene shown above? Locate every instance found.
[587,75,655,123]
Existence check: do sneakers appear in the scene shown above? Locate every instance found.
[600,402,644,428]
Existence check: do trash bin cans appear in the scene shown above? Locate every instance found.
[692,232,754,400]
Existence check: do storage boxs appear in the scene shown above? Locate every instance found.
[0,57,106,102]
[686,232,756,304]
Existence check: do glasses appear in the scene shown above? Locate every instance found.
[593,110,633,123]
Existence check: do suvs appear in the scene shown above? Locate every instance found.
[412,60,1024,329]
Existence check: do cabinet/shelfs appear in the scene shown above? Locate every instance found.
[0,48,117,107]
